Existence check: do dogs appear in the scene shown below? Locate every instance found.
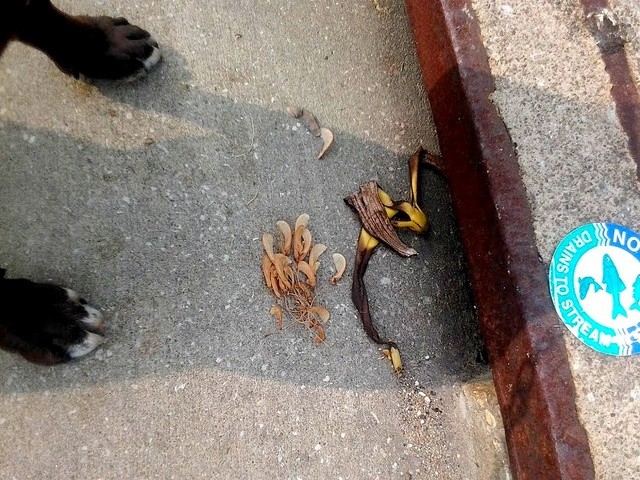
[0,1,162,367]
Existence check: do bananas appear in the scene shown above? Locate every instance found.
[343,147,446,374]
